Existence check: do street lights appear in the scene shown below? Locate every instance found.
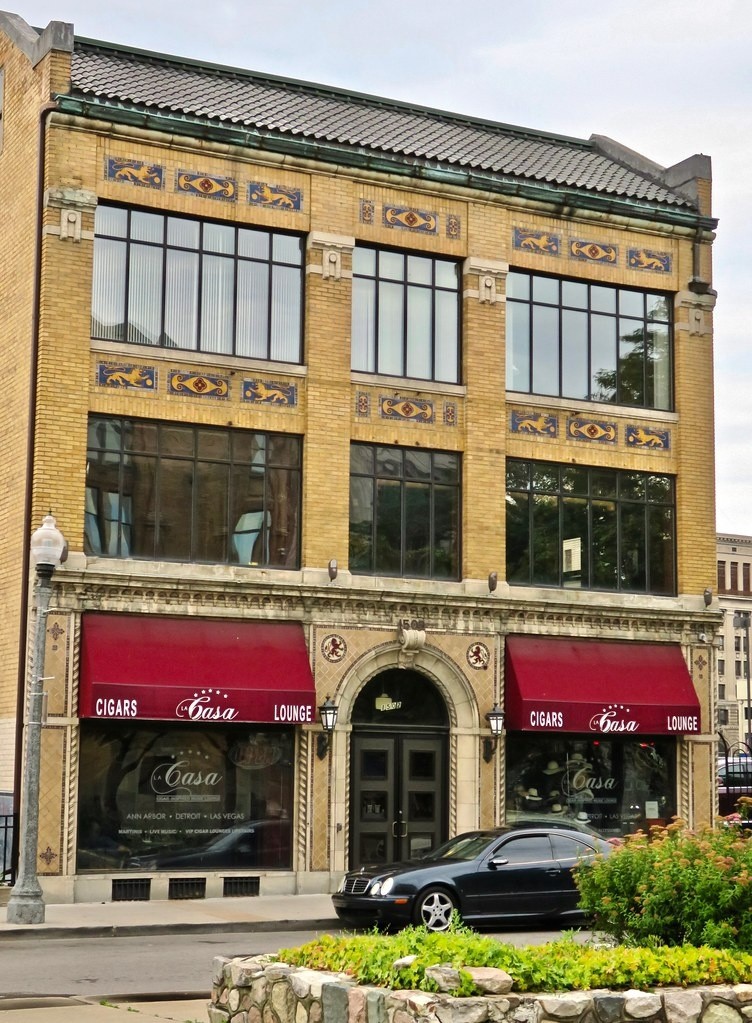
[7,513,65,923]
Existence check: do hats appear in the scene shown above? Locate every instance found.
[543,761,564,775]
[548,804,567,816]
[527,788,542,800]
[579,789,594,800]
[512,784,527,796]
[568,753,586,764]
[574,812,591,825]
[548,791,560,800]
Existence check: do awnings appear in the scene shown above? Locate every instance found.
[504,633,702,736]
[75,610,318,725]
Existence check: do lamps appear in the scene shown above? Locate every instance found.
[702,586,714,610]
[488,570,498,593]
[315,692,339,761]
[482,702,506,765]
[328,559,338,583]
[59,540,69,565]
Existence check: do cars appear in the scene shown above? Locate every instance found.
[717,757,752,831]
[129,816,293,877]
[507,811,608,843]
[331,821,620,937]
